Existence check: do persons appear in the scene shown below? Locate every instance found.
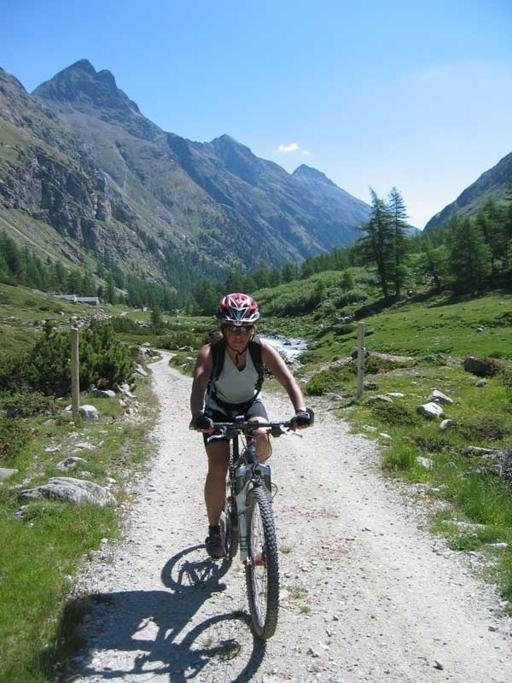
[190,292,310,559]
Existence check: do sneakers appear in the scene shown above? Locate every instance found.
[206,526,224,558]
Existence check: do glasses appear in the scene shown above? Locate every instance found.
[224,325,253,332]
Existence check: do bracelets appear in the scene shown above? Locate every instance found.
[296,407,307,412]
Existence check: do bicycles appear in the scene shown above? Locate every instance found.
[190,414,315,641]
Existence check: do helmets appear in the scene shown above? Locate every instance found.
[218,293,260,327]
